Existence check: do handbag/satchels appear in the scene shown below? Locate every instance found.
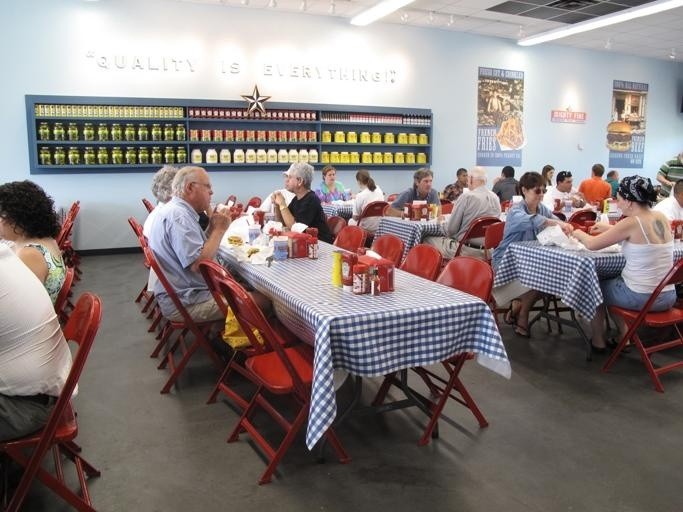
[222,304,264,351]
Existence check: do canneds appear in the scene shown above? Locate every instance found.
[39,146,187,165]
[37,121,186,141]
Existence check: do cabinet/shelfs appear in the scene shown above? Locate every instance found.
[23,94,432,174]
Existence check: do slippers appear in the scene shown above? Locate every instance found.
[590,338,632,353]
[504,300,529,337]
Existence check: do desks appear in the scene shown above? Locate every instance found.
[197,205,515,464]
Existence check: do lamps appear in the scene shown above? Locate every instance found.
[217,1,335,14]
[667,48,678,60]
[399,9,525,37]
[602,39,612,50]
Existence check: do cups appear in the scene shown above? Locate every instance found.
[564,201,572,212]
[227,234,243,253]
[343,188,351,201]
[264,213,275,222]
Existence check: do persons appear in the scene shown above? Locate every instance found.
[488,90,501,126]
[147,166,273,323]
[0,179,66,306]
[260,151,683,355]
[0,243,78,492]
[143,166,223,341]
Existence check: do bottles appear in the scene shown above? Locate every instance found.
[332,247,394,296]
[248,224,318,260]
[583,220,594,236]
[402,112,430,126]
[504,201,509,213]
[190,146,319,165]
[553,198,560,211]
[400,203,438,221]
[252,210,263,227]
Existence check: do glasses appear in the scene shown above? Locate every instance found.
[535,189,547,193]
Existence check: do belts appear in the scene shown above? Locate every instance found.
[463,243,486,249]
[20,394,58,405]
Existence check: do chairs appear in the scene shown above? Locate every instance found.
[124,193,221,398]
[325,225,498,448]
[603,258,678,396]
[23,189,86,344]
[218,275,358,486]
[2,291,104,510]
[323,191,682,354]
